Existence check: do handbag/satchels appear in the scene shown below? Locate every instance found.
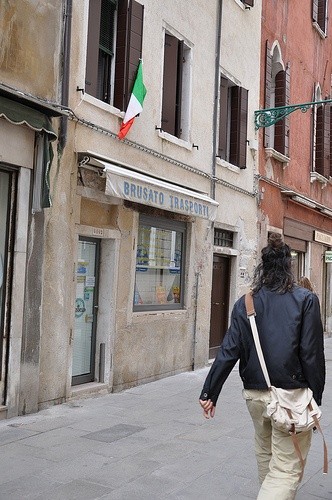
[266,386,328,483]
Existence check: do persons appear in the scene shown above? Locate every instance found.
[199,233,326,500]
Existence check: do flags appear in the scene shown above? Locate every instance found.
[117,58,147,140]
